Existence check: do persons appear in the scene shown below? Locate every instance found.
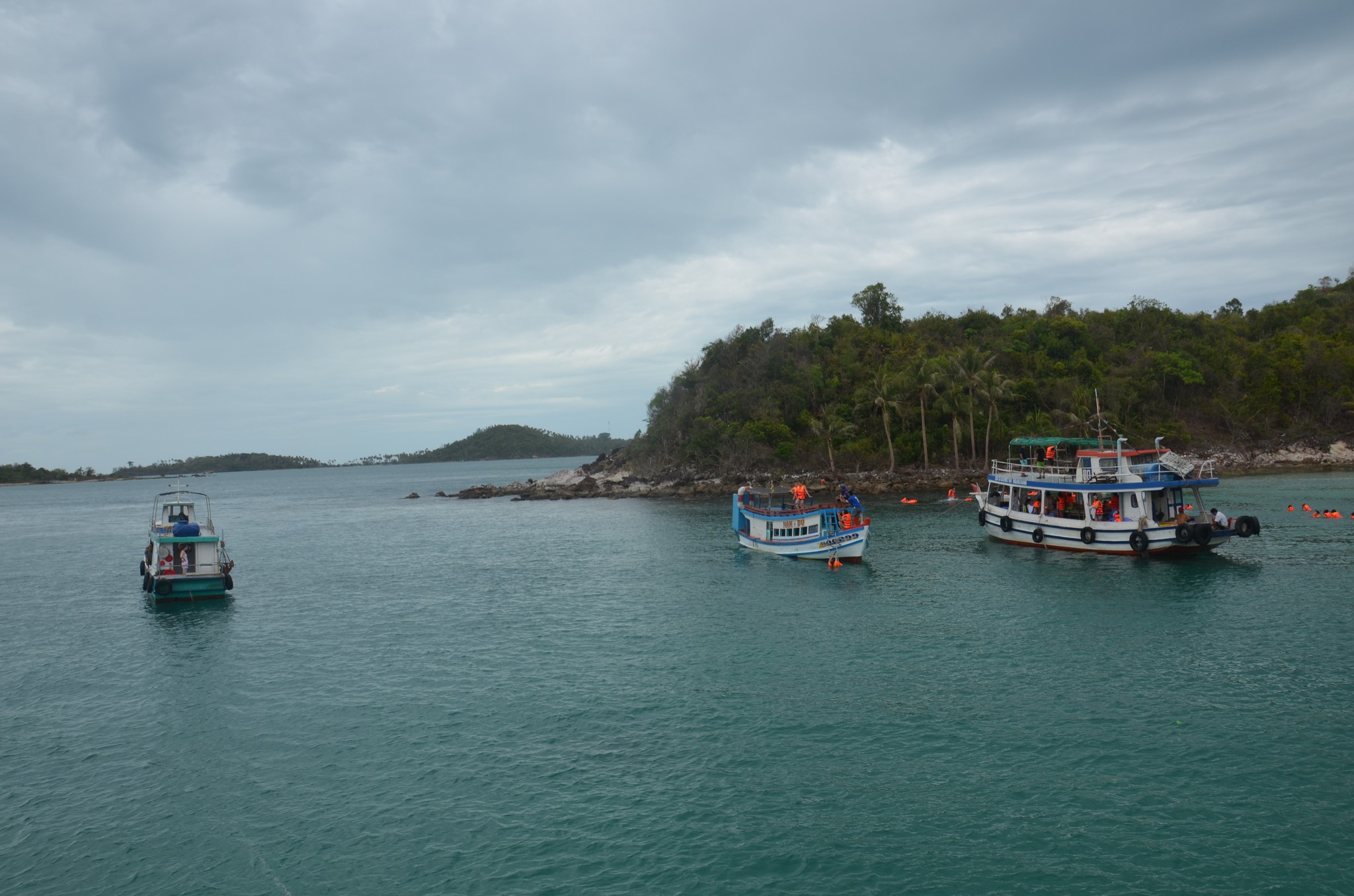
[1186,504,1189,507]
[1174,507,1195,525]
[906,497,910,501]
[837,510,852,532]
[840,490,861,526]
[836,484,850,502]
[789,480,801,505]
[1019,446,1065,479]
[948,486,955,497]
[793,480,812,515]
[1087,496,1103,521]
[1211,508,1228,530]
[1068,462,1077,475]
[1104,492,1118,514]
[1024,492,1076,518]
[180,545,188,574]
[1288,504,1354,519]
[1013,490,1021,511]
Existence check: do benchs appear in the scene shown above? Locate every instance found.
[1067,508,1085,521]
[784,502,811,510]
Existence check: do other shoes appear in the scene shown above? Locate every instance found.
[1025,475,1028,476]
[1214,528,1220,530]
[1021,475,1026,477]
[1036,476,1042,479]
[1042,476,1045,478]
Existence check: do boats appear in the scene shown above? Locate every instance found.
[137,475,234,600]
[981,388,1260,561]
[730,478,873,564]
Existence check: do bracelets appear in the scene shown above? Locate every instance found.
[841,500,842,501]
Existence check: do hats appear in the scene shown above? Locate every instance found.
[1093,495,1098,499]
[1178,507,1183,512]
[838,484,845,488]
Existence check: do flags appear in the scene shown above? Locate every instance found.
[770,481,774,494]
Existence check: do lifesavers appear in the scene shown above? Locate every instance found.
[1032,529,1043,543]
[979,511,985,526]
[226,574,233,590]
[1129,531,1149,552]
[1000,516,1012,532]
[1175,523,1193,543]
[143,572,149,591]
[1235,515,1253,538]
[140,560,145,576]
[985,491,1000,504]
[1193,524,1212,546]
[155,579,173,595]
[147,577,154,593]
[1080,527,1095,544]
[1250,516,1260,535]
